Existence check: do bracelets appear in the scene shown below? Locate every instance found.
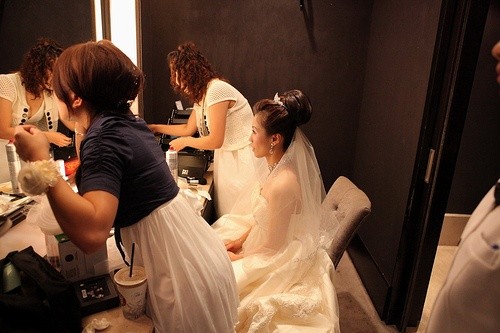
[17,160,58,196]
[75,129,84,136]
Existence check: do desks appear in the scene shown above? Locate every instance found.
[0,162,213,333]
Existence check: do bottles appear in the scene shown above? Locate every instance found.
[165,146,178,180]
[60,255,81,282]
[5,139,22,193]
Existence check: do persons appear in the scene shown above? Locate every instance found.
[425,36,500,333]
[223,89,341,333]
[13,41,237,333]
[0,37,75,149]
[146,43,268,217]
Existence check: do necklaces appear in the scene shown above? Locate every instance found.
[258,160,278,195]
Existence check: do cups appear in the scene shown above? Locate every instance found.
[114,268,148,321]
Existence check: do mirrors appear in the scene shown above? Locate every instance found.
[0,0,97,229]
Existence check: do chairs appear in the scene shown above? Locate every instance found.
[322,176,372,270]
